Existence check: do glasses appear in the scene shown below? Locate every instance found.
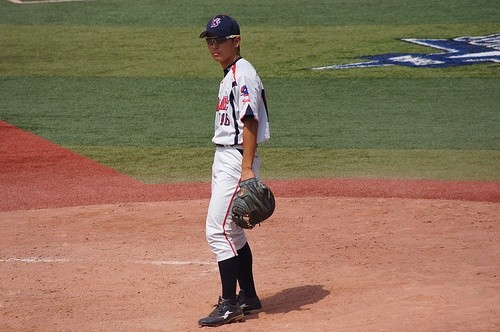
[204,34,241,44]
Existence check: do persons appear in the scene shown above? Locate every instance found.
[197,15,276,328]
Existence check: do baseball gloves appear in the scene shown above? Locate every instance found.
[231,178,276,230]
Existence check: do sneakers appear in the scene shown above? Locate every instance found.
[198,296,245,326]
[235,292,262,315]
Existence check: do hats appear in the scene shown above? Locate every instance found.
[199,15,240,38]
